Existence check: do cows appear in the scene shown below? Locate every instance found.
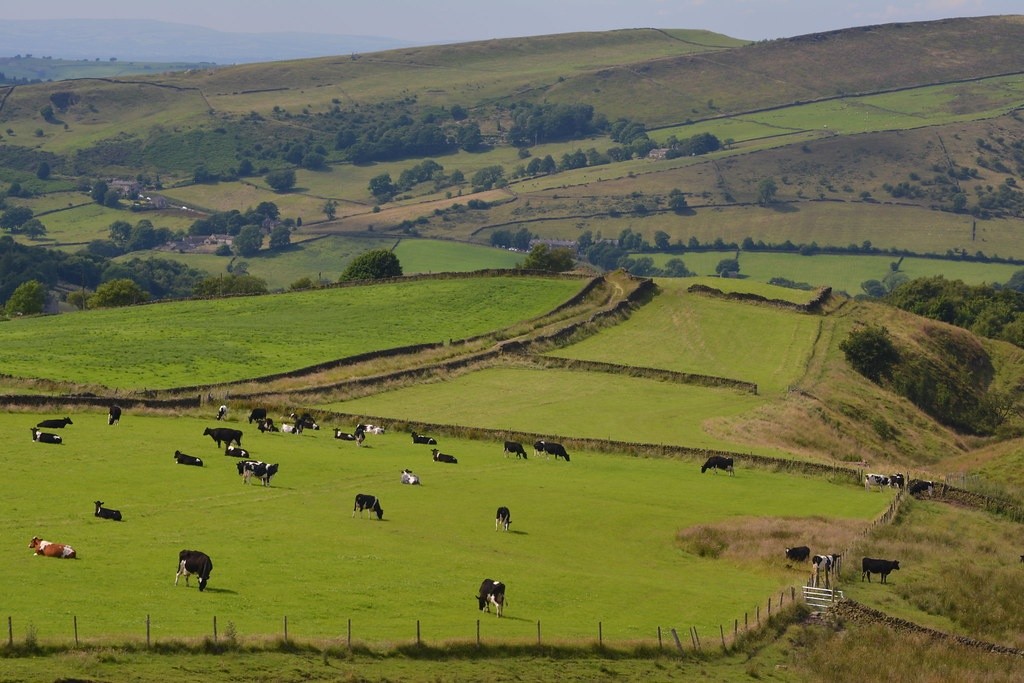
[108,406,122,425]
[701,456,735,477]
[175,549,212,591]
[352,494,384,520]
[30,416,73,444]
[216,405,227,421]
[475,579,505,618]
[94,501,122,521]
[785,546,900,584]
[237,460,279,487]
[431,449,457,463]
[28,536,76,558]
[174,450,203,466]
[864,473,933,499]
[248,408,385,446]
[203,427,249,458]
[496,506,512,532]
[411,432,437,444]
[503,440,570,461]
[400,469,420,484]
